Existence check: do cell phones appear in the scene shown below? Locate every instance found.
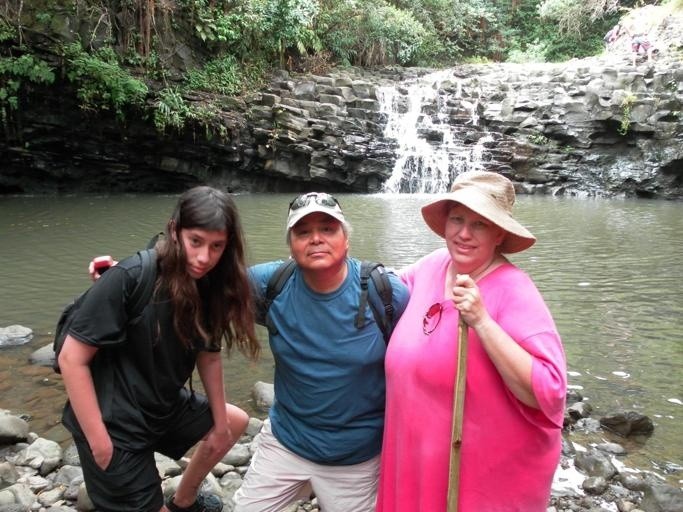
[94,255,113,274]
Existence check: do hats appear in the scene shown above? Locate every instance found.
[420,170,537,254]
[287,191,346,230]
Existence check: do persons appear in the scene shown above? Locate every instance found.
[53,185,256,511]
[82,191,413,512]
[379,171,568,512]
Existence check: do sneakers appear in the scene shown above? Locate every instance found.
[165,493,224,512]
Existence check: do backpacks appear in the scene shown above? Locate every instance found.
[52,249,158,376]
